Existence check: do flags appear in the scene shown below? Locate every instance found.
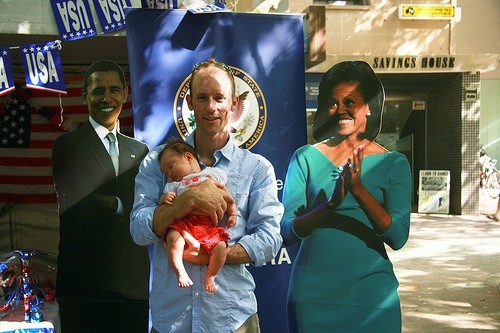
[0,63,134,203]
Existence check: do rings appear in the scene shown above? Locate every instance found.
[353,167,360,174]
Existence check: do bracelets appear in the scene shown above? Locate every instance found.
[328,197,336,208]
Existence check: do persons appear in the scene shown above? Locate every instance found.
[280,60,413,333]
[158,142,239,293]
[52,60,149,333]
[129,59,285,333]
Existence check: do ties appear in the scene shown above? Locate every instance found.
[107,133,119,182]
